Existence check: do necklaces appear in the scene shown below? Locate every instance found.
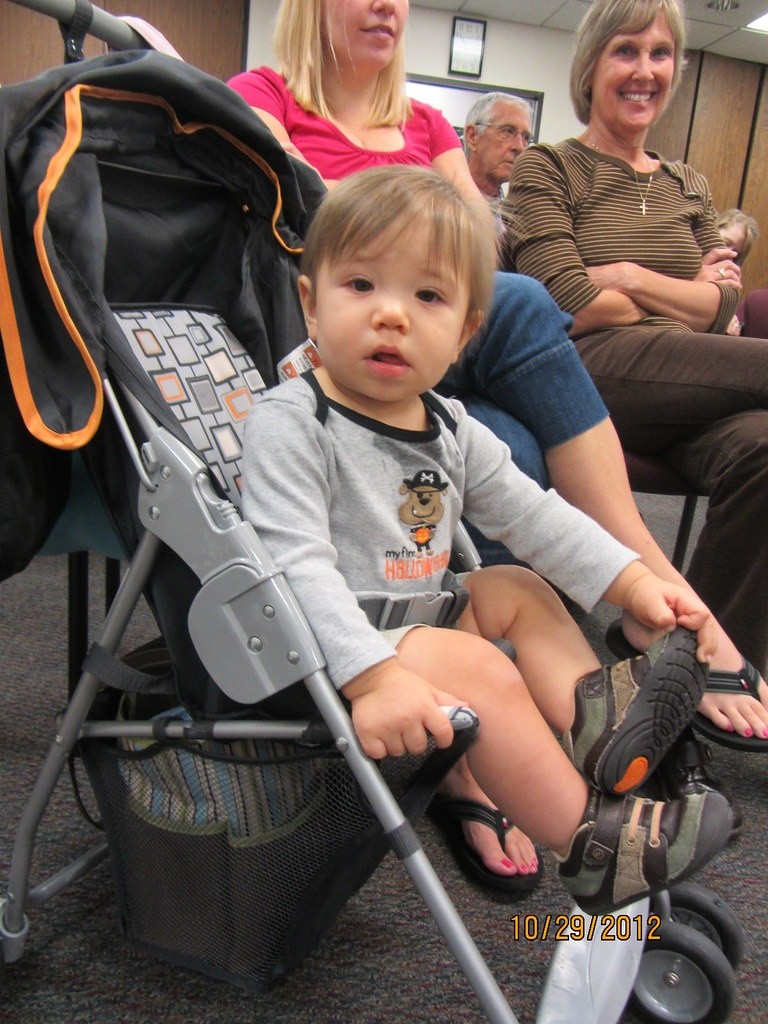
[590,141,654,215]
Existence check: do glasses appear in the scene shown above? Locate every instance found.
[473,121,534,145]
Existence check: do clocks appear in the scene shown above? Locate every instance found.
[448,16,487,77]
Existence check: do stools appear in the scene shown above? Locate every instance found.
[606,446,711,661]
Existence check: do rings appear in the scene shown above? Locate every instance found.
[718,270,726,277]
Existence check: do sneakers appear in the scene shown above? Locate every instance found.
[544,783,735,918]
[559,619,709,793]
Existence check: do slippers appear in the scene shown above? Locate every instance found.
[604,615,767,753]
[427,788,542,899]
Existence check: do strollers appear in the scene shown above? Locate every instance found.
[0,0,747,1024]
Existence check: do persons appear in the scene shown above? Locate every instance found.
[495,1,767,687]
[463,93,533,233]
[715,209,758,268]
[237,164,744,921]
[225,0,768,903]
